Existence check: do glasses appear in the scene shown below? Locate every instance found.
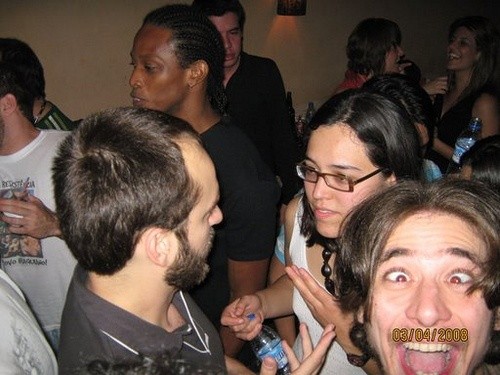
[296,160,386,192]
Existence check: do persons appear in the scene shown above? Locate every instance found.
[221,15,500,375]
[52,106,336,375]
[190,0,302,230]
[0,38,87,375]
[128,4,279,375]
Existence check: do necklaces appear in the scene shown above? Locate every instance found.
[34,101,46,122]
[322,245,335,295]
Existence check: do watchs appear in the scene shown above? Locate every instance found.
[346,353,370,368]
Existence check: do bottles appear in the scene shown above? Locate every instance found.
[247,312,293,375]
[296,116,304,138]
[286,92,295,125]
[445,117,483,174]
[306,103,316,124]
